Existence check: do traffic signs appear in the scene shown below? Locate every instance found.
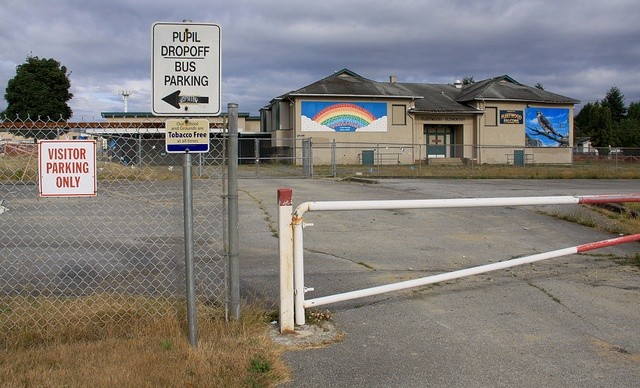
[151,21,221,116]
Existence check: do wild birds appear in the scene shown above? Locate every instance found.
[536,110,564,140]
